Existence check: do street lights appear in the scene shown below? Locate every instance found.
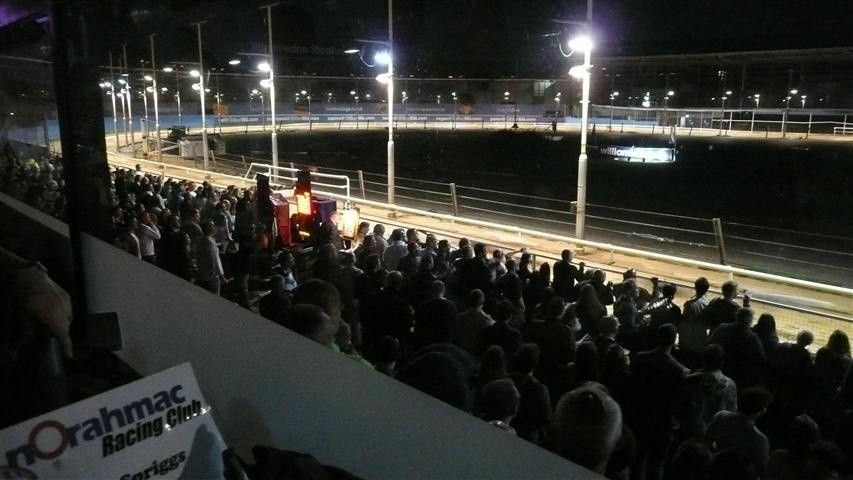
[402,93,409,126]
[503,93,517,127]
[783,89,813,139]
[99,67,182,164]
[351,91,360,129]
[551,92,562,131]
[229,57,279,186]
[188,68,223,175]
[346,45,396,221]
[608,91,735,130]
[544,31,593,253]
[452,92,459,129]
[252,89,266,131]
[302,90,314,131]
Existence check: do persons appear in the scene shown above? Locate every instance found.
[112,163,376,371]
[0,146,68,223]
[354,222,851,480]
